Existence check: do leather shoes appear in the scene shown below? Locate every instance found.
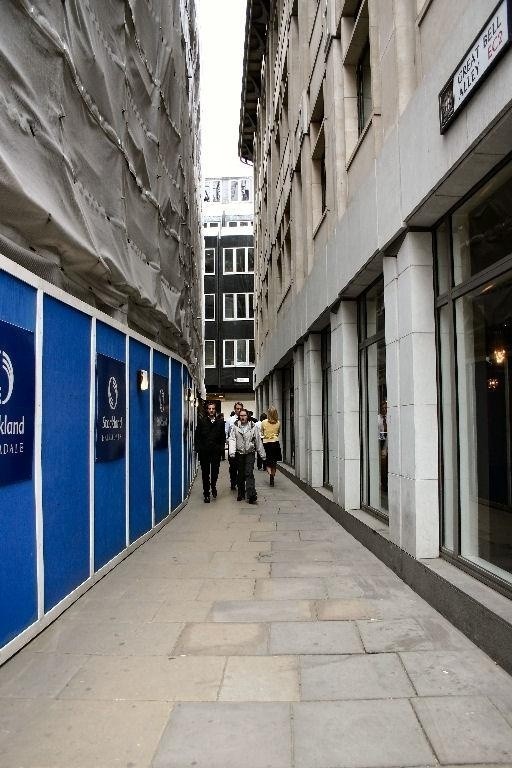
[269,473,275,487]
[202,496,259,504]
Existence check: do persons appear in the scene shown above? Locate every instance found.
[378,400,387,447]
[194,400,282,505]
